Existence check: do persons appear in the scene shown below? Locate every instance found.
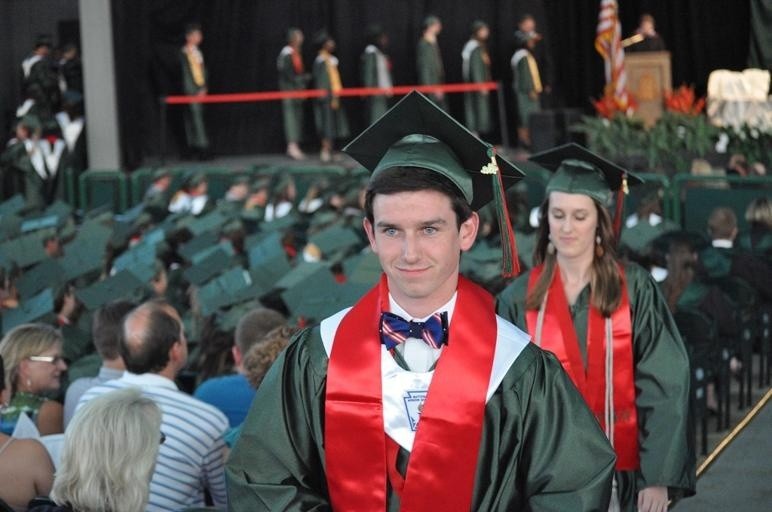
[1,1,771,511]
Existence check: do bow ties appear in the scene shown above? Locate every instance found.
[377,308,450,352]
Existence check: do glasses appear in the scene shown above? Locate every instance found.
[28,353,67,365]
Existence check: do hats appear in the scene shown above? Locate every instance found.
[635,176,665,203]
[460,219,542,288]
[0,160,391,332]
[528,141,646,208]
[341,89,527,213]
[618,210,678,259]
